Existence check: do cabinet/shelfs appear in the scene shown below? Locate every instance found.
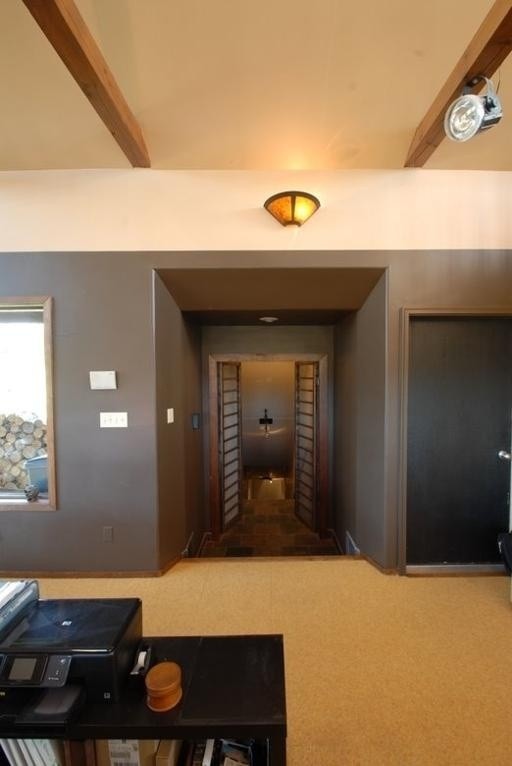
[0,634,289,766]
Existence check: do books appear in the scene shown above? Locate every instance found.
[177,738,270,766]
[0,738,64,766]
[0,580,38,631]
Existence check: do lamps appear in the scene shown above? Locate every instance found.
[264,190,322,226]
[442,74,501,142]
